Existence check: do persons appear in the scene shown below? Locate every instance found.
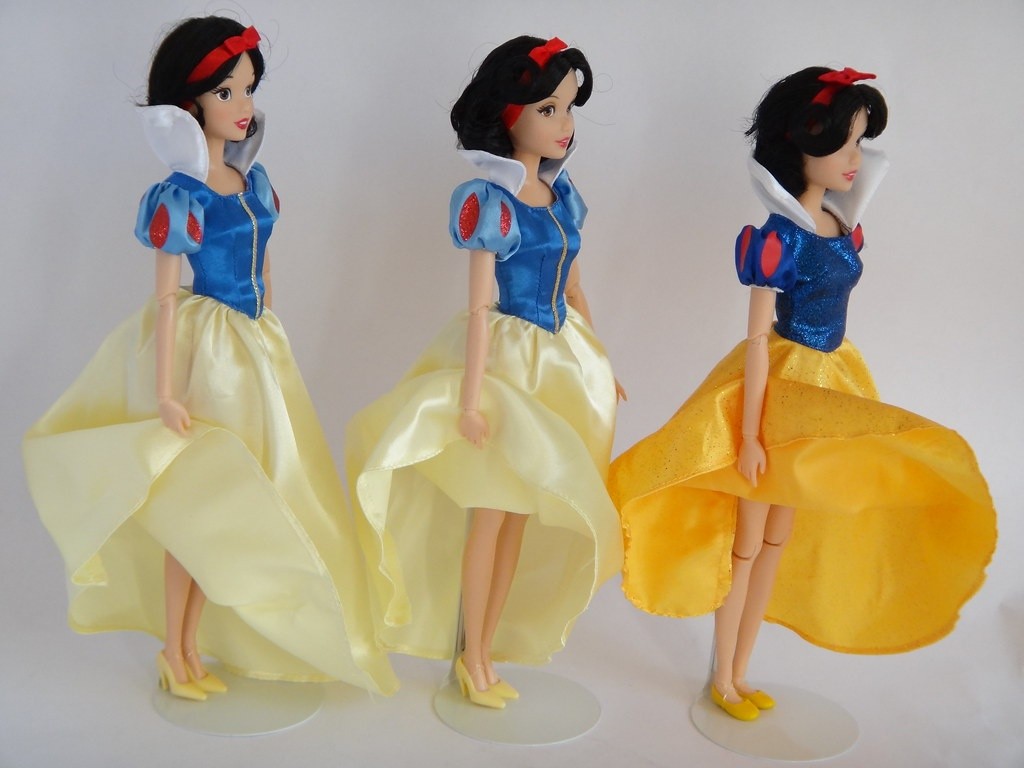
[346,35,625,707]
[609,64,998,724]
[22,15,402,701]
